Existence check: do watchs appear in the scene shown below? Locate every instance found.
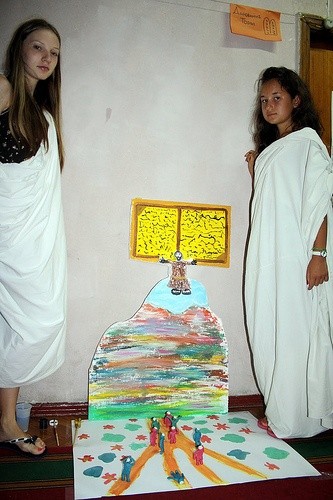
[313,251,326,257]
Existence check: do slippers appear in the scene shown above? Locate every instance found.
[0,433,48,459]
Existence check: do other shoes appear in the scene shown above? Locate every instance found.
[267,427,333,442]
[257,416,269,430]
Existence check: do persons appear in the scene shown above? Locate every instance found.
[0,19,67,454]
[242,67,332,439]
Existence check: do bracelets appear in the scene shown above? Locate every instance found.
[311,248,326,250]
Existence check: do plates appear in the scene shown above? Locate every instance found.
[16,403,32,433]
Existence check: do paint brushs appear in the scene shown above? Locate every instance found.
[53,418,59,446]
